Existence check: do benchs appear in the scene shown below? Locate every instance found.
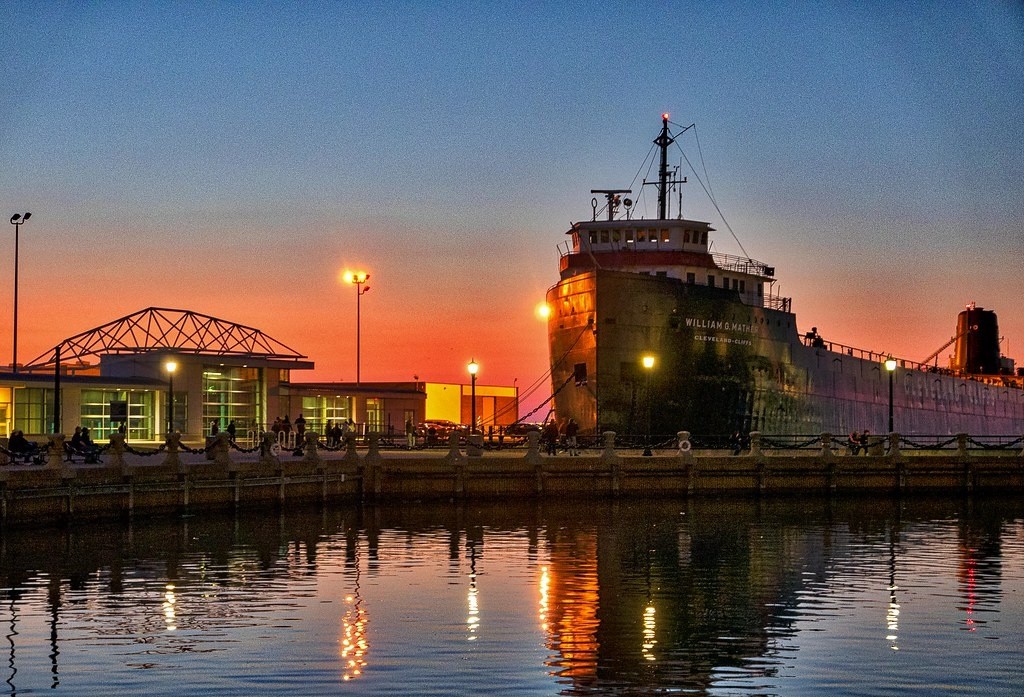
[7,444,49,465]
[64,440,103,465]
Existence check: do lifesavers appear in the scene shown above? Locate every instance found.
[678,440,691,452]
[269,443,282,457]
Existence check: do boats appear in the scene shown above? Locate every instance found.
[546,111,1024,445]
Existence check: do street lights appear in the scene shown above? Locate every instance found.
[642,354,656,457]
[343,272,371,388]
[884,358,897,453]
[469,357,479,436]
[9,212,33,373]
[166,361,178,434]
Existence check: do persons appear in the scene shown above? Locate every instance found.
[8,430,36,463]
[227,421,236,445]
[272,415,292,443]
[728,430,742,455]
[406,420,413,446]
[295,414,306,435]
[71,427,99,451]
[542,419,558,456]
[559,417,579,456]
[860,430,872,454]
[325,418,356,448]
[848,430,862,455]
[212,422,218,435]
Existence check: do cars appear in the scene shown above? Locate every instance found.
[505,423,544,435]
